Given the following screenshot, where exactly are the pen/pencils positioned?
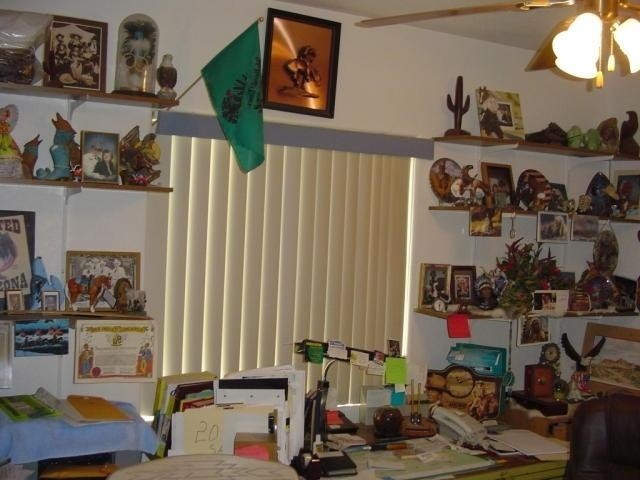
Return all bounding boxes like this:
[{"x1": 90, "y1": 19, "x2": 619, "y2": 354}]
[
  {"x1": 417, "y1": 382, "x2": 422, "y2": 423},
  {"x1": 411, "y1": 379, "x2": 415, "y2": 423}
]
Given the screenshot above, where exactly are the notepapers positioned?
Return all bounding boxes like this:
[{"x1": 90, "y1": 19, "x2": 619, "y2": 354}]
[
  {"x1": 307, "y1": 344, "x2": 324, "y2": 364},
  {"x1": 384, "y1": 389, "x2": 392, "y2": 407},
  {"x1": 365, "y1": 390, "x2": 385, "y2": 408},
  {"x1": 368, "y1": 361, "x2": 385, "y2": 376},
  {"x1": 374, "y1": 351, "x2": 385, "y2": 367},
  {"x1": 446, "y1": 314, "x2": 471, "y2": 339},
  {"x1": 327, "y1": 410, "x2": 345, "y2": 426},
  {"x1": 233, "y1": 444, "x2": 270, "y2": 463},
  {"x1": 392, "y1": 387, "x2": 405, "y2": 406},
  {"x1": 350, "y1": 350, "x2": 369, "y2": 368},
  {"x1": 385, "y1": 357, "x2": 411, "y2": 384},
  {"x1": 395, "y1": 384, "x2": 406, "y2": 393},
  {"x1": 328, "y1": 340, "x2": 348, "y2": 360}
]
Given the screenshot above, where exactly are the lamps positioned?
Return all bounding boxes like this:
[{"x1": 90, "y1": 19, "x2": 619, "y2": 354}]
[
  {"x1": 550, "y1": 13, "x2": 605, "y2": 89},
  {"x1": 608, "y1": 18, "x2": 640, "y2": 77}
]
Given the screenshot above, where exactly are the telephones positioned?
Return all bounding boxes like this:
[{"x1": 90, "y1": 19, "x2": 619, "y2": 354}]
[{"x1": 431, "y1": 404, "x2": 488, "y2": 446}]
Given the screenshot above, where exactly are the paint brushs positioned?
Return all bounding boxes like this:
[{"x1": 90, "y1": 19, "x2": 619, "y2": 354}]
[{"x1": 363, "y1": 442, "x2": 407, "y2": 452}]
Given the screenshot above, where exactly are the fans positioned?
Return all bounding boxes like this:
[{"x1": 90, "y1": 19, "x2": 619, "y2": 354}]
[{"x1": 353, "y1": 0, "x2": 640, "y2": 72}]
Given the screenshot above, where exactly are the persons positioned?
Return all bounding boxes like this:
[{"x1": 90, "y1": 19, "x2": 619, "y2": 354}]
[{"x1": 83, "y1": 142, "x2": 115, "y2": 182}]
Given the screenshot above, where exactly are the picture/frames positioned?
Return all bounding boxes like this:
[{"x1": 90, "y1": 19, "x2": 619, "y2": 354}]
[
  {"x1": 580, "y1": 322, "x2": 640, "y2": 395},
  {"x1": 81, "y1": 128, "x2": 120, "y2": 185},
  {"x1": 41, "y1": 15, "x2": 108, "y2": 94},
  {"x1": 469, "y1": 206, "x2": 502, "y2": 237},
  {"x1": 262, "y1": 6, "x2": 342, "y2": 119},
  {"x1": 419, "y1": 263, "x2": 451, "y2": 308},
  {"x1": 536, "y1": 211, "x2": 569, "y2": 244},
  {"x1": 450, "y1": 265, "x2": 477, "y2": 304},
  {"x1": 516, "y1": 313, "x2": 550, "y2": 347},
  {"x1": 475, "y1": 88, "x2": 525, "y2": 146},
  {"x1": 65, "y1": 250, "x2": 141, "y2": 313},
  {"x1": 481, "y1": 161, "x2": 515, "y2": 208},
  {"x1": 613, "y1": 169, "x2": 640, "y2": 220},
  {"x1": 531, "y1": 289, "x2": 569, "y2": 315}
]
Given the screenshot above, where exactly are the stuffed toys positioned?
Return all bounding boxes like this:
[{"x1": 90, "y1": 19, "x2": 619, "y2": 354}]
[{"x1": 65, "y1": 274, "x2": 112, "y2": 312}]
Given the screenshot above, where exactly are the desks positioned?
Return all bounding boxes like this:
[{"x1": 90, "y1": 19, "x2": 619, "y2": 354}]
[
  {"x1": 0, "y1": 400, "x2": 161, "y2": 480},
  {"x1": 301, "y1": 423, "x2": 569, "y2": 479}
]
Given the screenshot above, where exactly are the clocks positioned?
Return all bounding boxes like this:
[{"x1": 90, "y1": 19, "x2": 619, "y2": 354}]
[
  {"x1": 541, "y1": 343, "x2": 561, "y2": 362},
  {"x1": 424, "y1": 364, "x2": 502, "y2": 420}
]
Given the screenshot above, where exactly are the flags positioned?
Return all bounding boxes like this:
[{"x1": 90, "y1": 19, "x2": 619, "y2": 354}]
[{"x1": 200, "y1": 16, "x2": 266, "y2": 174}]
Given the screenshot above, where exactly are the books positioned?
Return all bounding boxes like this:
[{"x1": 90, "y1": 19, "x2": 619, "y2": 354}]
[
  {"x1": 145, "y1": 364, "x2": 307, "y2": 465},
  {"x1": 315, "y1": 451, "x2": 358, "y2": 477}
]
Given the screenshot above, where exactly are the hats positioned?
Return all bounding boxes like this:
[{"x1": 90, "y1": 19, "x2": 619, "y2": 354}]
[
  {"x1": 71, "y1": 30, "x2": 83, "y2": 39},
  {"x1": 112, "y1": 260, "x2": 122, "y2": 265},
  {"x1": 56, "y1": 33, "x2": 64, "y2": 39},
  {"x1": 91, "y1": 257, "x2": 99, "y2": 262},
  {"x1": 99, "y1": 261, "x2": 107, "y2": 266},
  {"x1": 57, "y1": 43, "x2": 67, "y2": 48}
]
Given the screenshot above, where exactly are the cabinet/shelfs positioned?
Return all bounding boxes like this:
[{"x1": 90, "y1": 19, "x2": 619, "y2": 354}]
[
  {"x1": 0, "y1": 313, "x2": 154, "y2": 322},
  {"x1": 0, "y1": 178, "x2": 173, "y2": 193},
  {"x1": 432, "y1": 136, "x2": 613, "y2": 158},
  {"x1": 413, "y1": 308, "x2": 490, "y2": 320},
  {"x1": 0, "y1": 81, "x2": 180, "y2": 120},
  {"x1": 429, "y1": 205, "x2": 612, "y2": 220}
]
